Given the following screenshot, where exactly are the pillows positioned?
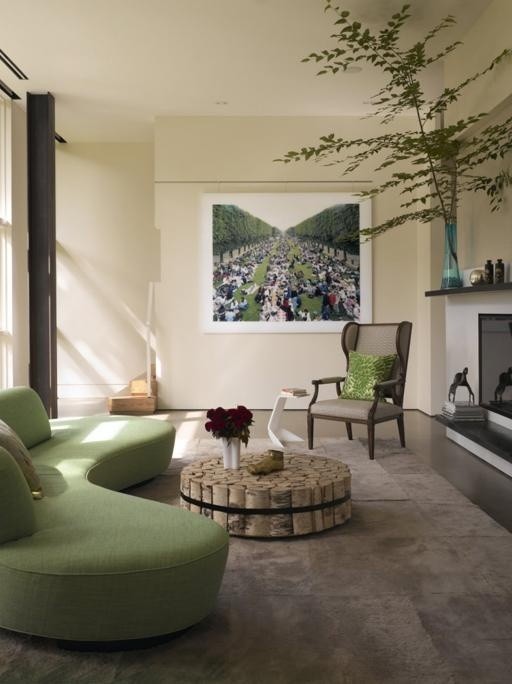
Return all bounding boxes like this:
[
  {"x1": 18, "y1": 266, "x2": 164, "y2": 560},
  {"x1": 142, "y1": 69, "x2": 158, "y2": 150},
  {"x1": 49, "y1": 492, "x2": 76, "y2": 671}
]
[
  {"x1": 2, "y1": 419, "x2": 45, "y2": 548},
  {"x1": 338, "y1": 349, "x2": 396, "y2": 403}
]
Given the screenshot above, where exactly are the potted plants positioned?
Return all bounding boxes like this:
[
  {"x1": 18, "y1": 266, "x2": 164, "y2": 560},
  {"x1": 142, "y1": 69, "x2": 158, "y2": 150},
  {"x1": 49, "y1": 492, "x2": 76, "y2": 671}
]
[{"x1": 270, "y1": 0, "x2": 512, "y2": 291}]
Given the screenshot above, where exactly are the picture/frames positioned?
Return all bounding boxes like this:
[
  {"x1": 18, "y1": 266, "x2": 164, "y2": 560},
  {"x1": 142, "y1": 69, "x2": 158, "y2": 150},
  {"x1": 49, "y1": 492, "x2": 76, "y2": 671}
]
[{"x1": 199, "y1": 191, "x2": 374, "y2": 334}]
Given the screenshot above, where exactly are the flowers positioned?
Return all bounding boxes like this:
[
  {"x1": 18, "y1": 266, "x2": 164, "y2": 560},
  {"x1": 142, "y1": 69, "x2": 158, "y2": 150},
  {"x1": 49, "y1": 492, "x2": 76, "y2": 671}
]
[{"x1": 205, "y1": 405, "x2": 254, "y2": 447}]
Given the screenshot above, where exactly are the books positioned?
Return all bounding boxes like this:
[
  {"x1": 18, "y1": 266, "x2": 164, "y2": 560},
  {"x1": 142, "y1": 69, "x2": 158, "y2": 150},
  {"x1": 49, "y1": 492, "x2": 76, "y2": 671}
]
[{"x1": 441, "y1": 401, "x2": 487, "y2": 422}]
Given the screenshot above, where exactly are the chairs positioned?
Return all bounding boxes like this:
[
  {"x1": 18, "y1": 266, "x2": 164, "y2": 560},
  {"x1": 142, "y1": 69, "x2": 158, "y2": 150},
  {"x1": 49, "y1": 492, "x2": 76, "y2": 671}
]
[{"x1": 306, "y1": 320, "x2": 412, "y2": 460}]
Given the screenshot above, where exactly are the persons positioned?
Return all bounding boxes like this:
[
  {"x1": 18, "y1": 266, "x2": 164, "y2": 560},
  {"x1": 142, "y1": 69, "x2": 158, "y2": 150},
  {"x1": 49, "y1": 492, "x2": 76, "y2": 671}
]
[{"x1": 213, "y1": 238, "x2": 360, "y2": 321}]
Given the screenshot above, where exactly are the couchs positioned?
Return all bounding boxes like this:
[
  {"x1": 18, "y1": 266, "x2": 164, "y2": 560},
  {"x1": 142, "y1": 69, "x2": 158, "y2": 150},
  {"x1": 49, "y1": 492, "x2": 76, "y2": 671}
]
[{"x1": 1, "y1": 383, "x2": 231, "y2": 651}]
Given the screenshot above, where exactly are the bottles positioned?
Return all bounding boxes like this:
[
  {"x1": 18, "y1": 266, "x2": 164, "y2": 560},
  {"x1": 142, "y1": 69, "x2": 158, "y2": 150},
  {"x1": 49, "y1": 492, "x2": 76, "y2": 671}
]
[
  {"x1": 495, "y1": 259, "x2": 504, "y2": 284},
  {"x1": 485, "y1": 260, "x2": 493, "y2": 284}
]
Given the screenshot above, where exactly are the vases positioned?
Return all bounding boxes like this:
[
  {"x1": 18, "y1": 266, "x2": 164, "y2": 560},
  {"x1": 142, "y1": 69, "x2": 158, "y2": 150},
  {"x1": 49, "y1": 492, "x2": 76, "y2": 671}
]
[{"x1": 222, "y1": 437, "x2": 240, "y2": 469}]
